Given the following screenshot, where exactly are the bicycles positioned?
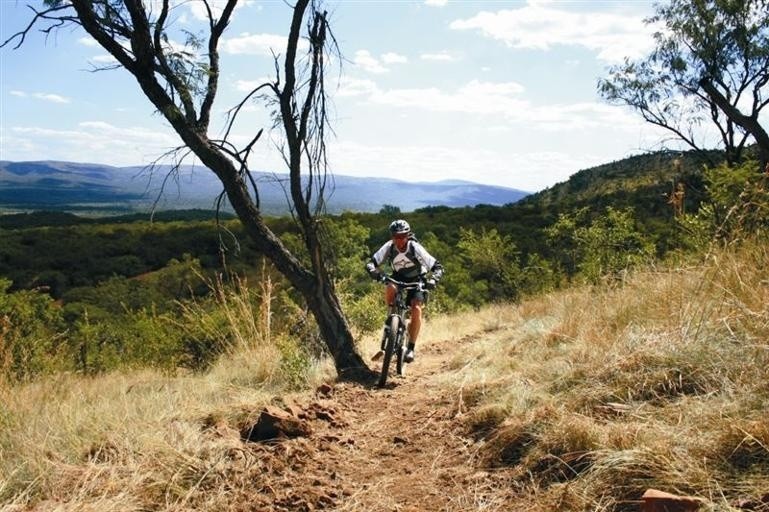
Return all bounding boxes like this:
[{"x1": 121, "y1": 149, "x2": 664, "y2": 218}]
[{"x1": 367, "y1": 270, "x2": 439, "y2": 387}]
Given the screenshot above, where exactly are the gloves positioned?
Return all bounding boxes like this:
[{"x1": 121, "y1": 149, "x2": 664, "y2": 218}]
[{"x1": 425, "y1": 278, "x2": 436, "y2": 290}]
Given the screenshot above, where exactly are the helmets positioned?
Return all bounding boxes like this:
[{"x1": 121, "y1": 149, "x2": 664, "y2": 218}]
[{"x1": 389, "y1": 220, "x2": 411, "y2": 235}]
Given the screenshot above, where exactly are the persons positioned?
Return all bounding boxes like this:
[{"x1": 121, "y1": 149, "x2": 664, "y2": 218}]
[{"x1": 365, "y1": 219, "x2": 445, "y2": 364}]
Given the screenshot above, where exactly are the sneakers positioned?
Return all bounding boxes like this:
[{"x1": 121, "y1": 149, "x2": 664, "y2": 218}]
[
  {"x1": 370, "y1": 270, "x2": 381, "y2": 280},
  {"x1": 404, "y1": 350, "x2": 414, "y2": 364}
]
